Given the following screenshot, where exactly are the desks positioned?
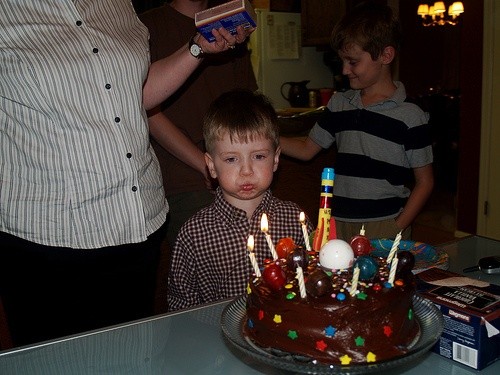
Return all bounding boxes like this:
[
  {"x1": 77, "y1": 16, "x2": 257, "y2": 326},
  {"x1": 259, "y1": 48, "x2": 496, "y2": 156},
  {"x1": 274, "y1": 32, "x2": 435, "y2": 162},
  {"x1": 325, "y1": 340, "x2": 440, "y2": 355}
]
[
  {"x1": 277, "y1": 110, "x2": 334, "y2": 226},
  {"x1": 0, "y1": 234, "x2": 500, "y2": 375}
]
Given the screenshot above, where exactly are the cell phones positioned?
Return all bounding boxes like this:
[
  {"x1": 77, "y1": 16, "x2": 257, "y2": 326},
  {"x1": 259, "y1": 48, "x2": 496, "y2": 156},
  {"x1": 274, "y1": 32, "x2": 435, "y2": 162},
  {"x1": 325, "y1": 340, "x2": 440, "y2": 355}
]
[{"x1": 463, "y1": 256, "x2": 500, "y2": 275}]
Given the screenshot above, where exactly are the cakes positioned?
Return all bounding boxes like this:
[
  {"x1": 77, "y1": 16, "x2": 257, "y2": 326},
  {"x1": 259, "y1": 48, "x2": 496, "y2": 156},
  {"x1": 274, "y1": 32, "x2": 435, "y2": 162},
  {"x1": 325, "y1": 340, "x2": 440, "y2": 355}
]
[{"x1": 244, "y1": 218, "x2": 419, "y2": 366}]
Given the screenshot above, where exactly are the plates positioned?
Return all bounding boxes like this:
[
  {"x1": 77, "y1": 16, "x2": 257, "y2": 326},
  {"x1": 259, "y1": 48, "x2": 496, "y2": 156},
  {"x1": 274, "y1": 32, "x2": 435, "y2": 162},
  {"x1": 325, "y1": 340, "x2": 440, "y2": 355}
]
[
  {"x1": 220, "y1": 291, "x2": 444, "y2": 375},
  {"x1": 411, "y1": 245, "x2": 449, "y2": 274},
  {"x1": 371, "y1": 239, "x2": 436, "y2": 269}
]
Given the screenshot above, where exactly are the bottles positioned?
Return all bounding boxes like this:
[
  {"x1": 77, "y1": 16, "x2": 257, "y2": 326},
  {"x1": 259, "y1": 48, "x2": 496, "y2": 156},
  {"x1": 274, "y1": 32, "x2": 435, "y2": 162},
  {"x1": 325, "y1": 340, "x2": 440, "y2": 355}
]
[{"x1": 463, "y1": 256, "x2": 500, "y2": 275}]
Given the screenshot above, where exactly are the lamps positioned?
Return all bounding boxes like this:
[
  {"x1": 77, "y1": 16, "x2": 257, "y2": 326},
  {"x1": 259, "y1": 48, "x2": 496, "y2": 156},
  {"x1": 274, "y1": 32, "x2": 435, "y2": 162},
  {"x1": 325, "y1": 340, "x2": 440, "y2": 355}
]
[{"x1": 418, "y1": 0, "x2": 465, "y2": 27}]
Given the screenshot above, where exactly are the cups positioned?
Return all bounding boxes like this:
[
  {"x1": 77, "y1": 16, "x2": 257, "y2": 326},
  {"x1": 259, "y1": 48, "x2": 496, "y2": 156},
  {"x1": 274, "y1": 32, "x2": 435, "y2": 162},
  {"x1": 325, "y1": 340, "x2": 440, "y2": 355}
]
[
  {"x1": 319, "y1": 88, "x2": 333, "y2": 106},
  {"x1": 308, "y1": 89, "x2": 319, "y2": 107}
]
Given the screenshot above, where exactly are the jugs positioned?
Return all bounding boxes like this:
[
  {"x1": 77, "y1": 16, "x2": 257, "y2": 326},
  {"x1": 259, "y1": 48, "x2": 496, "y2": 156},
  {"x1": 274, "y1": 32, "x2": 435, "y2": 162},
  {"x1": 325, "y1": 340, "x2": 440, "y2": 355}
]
[{"x1": 280, "y1": 80, "x2": 311, "y2": 108}]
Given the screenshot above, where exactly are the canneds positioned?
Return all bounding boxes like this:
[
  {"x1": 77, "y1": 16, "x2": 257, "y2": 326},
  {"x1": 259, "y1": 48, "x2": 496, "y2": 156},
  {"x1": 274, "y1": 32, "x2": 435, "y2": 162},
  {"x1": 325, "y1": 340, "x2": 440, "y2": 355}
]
[{"x1": 309, "y1": 91, "x2": 316, "y2": 107}]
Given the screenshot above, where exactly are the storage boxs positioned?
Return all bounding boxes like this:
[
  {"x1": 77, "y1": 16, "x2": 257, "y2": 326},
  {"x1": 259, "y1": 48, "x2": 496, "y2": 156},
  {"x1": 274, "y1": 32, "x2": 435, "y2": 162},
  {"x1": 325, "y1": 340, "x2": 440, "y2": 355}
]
[
  {"x1": 416, "y1": 276, "x2": 500, "y2": 372},
  {"x1": 193, "y1": 0, "x2": 259, "y2": 40}
]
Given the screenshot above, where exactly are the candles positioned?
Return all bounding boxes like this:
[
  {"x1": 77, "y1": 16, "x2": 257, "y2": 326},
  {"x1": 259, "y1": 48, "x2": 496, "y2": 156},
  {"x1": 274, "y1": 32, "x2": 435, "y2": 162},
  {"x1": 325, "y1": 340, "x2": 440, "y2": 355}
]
[
  {"x1": 298, "y1": 210, "x2": 312, "y2": 252},
  {"x1": 349, "y1": 263, "x2": 360, "y2": 295},
  {"x1": 388, "y1": 252, "x2": 398, "y2": 283},
  {"x1": 247, "y1": 236, "x2": 261, "y2": 278},
  {"x1": 260, "y1": 213, "x2": 279, "y2": 260},
  {"x1": 293, "y1": 258, "x2": 307, "y2": 298},
  {"x1": 387, "y1": 230, "x2": 402, "y2": 263},
  {"x1": 359, "y1": 224, "x2": 367, "y2": 237}
]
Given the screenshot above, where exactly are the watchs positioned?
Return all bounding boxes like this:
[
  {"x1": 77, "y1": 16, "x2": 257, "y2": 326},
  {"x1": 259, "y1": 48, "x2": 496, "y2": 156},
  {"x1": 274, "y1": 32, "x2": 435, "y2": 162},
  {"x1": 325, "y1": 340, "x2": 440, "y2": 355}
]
[{"x1": 189, "y1": 33, "x2": 208, "y2": 58}]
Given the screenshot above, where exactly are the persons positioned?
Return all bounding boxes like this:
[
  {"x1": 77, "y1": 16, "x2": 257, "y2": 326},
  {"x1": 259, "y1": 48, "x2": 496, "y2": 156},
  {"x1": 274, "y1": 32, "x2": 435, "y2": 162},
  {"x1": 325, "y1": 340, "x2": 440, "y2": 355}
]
[
  {"x1": 167, "y1": 90, "x2": 315, "y2": 316},
  {"x1": 0, "y1": 0, "x2": 255, "y2": 346},
  {"x1": 139, "y1": 0, "x2": 258, "y2": 251},
  {"x1": 279, "y1": 5, "x2": 436, "y2": 244}
]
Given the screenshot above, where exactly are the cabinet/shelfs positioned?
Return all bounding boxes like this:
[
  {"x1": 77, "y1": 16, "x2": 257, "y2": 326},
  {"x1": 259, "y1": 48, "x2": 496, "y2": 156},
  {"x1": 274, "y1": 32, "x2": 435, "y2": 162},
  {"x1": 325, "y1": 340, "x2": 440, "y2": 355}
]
[{"x1": 270, "y1": 0, "x2": 399, "y2": 42}]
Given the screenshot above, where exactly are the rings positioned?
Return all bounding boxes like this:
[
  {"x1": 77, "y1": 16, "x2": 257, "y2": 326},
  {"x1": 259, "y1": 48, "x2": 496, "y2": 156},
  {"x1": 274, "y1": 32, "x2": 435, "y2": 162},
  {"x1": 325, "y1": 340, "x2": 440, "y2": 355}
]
[{"x1": 227, "y1": 42, "x2": 236, "y2": 49}]
